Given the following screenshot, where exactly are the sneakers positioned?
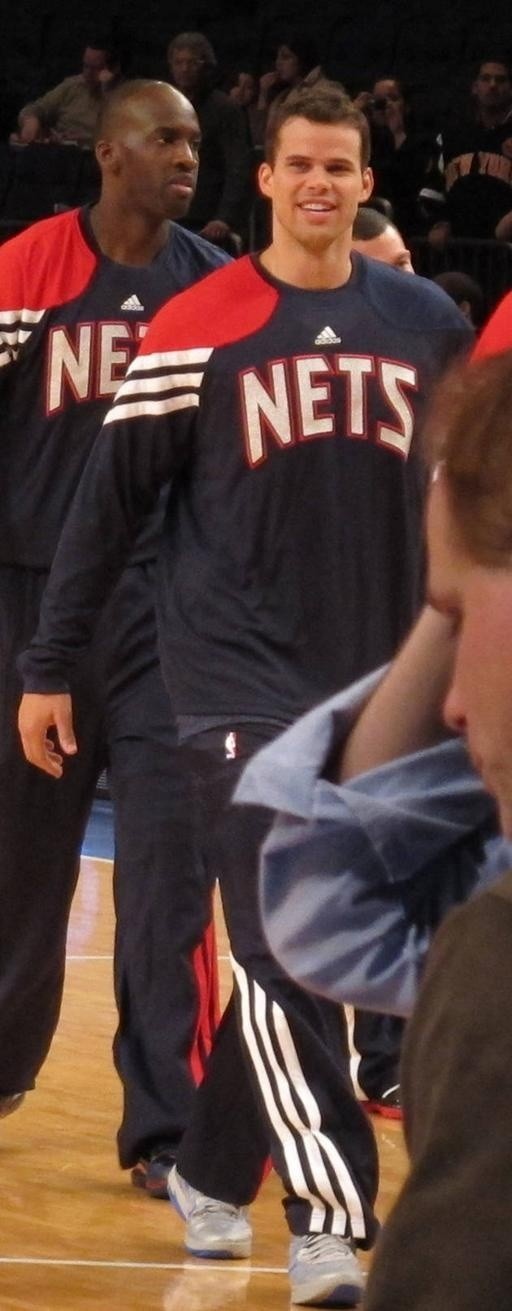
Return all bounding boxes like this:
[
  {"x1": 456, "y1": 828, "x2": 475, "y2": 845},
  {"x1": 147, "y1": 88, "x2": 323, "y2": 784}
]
[
  {"x1": 286, "y1": 1233, "x2": 364, "y2": 1307},
  {"x1": 360, "y1": 1078, "x2": 404, "y2": 1120},
  {"x1": 131, "y1": 1142, "x2": 176, "y2": 1200},
  {"x1": 165, "y1": 1163, "x2": 253, "y2": 1259}
]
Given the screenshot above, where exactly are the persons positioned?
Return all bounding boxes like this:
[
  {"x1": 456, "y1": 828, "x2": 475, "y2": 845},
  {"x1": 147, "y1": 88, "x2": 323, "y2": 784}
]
[
  {"x1": 0, "y1": 1, "x2": 512, "y2": 326},
  {"x1": 248, "y1": 570, "x2": 512, "y2": 1023},
  {"x1": 2, "y1": 71, "x2": 239, "y2": 1204},
  {"x1": 18, "y1": 81, "x2": 476, "y2": 1311},
  {"x1": 347, "y1": 341, "x2": 510, "y2": 1311}
]
